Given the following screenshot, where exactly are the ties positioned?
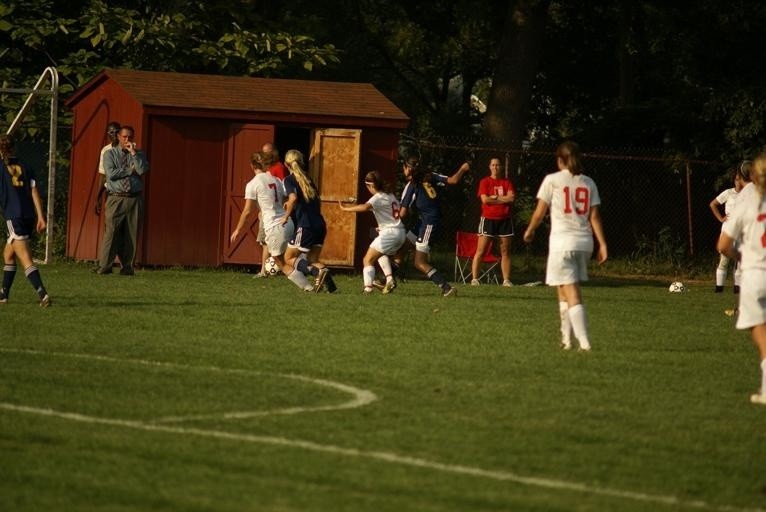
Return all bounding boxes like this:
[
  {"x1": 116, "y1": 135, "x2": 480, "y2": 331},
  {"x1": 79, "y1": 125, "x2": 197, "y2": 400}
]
[{"x1": 122, "y1": 151, "x2": 131, "y2": 192}]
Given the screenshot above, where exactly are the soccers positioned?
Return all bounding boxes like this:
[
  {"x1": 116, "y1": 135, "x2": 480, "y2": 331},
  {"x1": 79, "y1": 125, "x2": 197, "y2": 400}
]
[
  {"x1": 264, "y1": 257, "x2": 281, "y2": 276},
  {"x1": 669, "y1": 281, "x2": 685, "y2": 294}
]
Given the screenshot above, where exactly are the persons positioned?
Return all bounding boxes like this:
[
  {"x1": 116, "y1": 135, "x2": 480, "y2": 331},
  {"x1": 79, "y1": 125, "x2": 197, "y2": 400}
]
[
  {"x1": 281, "y1": 148, "x2": 338, "y2": 293},
  {"x1": 253, "y1": 143, "x2": 292, "y2": 280},
  {"x1": 92, "y1": 121, "x2": 123, "y2": 232},
  {"x1": 523, "y1": 141, "x2": 609, "y2": 352},
  {"x1": 338, "y1": 170, "x2": 408, "y2": 296},
  {"x1": 715, "y1": 159, "x2": 751, "y2": 301},
  {"x1": 1, "y1": 132, "x2": 53, "y2": 309},
  {"x1": 470, "y1": 154, "x2": 517, "y2": 287},
  {"x1": 388, "y1": 156, "x2": 473, "y2": 298},
  {"x1": 97, "y1": 125, "x2": 149, "y2": 276},
  {"x1": 733, "y1": 151, "x2": 766, "y2": 404},
  {"x1": 708, "y1": 162, "x2": 743, "y2": 295},
  {"x1": 228, "y1": 150, "x2": 316, "y2": 292}
]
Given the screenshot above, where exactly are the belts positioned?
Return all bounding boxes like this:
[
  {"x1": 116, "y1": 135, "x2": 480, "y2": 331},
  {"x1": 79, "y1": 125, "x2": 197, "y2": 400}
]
[{"x1": 109, "y1": 192, "x2": 135, "y2": 197}]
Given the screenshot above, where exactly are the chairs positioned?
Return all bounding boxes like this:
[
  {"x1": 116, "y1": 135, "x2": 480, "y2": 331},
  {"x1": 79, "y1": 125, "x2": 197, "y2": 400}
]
[{"x1": 454, "y1": 231, "x2": 499, "y2": 285}]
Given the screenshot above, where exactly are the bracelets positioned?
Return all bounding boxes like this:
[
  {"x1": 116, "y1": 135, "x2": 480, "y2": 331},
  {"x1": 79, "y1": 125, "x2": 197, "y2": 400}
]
[{"x1": 131, "y1": 153, "x2": 136, "y2": 157}]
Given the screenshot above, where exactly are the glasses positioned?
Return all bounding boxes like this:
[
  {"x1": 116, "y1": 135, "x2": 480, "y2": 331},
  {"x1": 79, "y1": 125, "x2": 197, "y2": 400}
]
[{"x1": 107, "y1": 130, "x2": 119, "y2": 135}]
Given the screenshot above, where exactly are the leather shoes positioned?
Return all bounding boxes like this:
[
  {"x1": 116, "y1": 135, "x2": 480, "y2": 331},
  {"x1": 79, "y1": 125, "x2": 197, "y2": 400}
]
[
  {"x1": 96, "y1": 267, "x2": 111, "y2": 275},
  {"x1": 120, "y1": 266, "x2": 133, "y2": 275}
]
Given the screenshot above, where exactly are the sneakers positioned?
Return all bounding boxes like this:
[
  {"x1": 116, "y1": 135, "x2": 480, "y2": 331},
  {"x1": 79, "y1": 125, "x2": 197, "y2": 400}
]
[
  {"x1": 39, "y1": 292, "x2": 50, "y2": 307},
  {"x1": 470, "y1": 279, "x2": 479, "y2": 286},
  {"x1": 502, "y1": 280, "x2": 512, "y2": 287},
  {"x1": 0, "y1": 287, "x2": 8, "y2": 304},
  {"x1": 441, "y1": 283, "x2": 456, "y2": 297},
  {"x1": 303, "y1": 267, "x2": 338, "y2": 294},
  {"x1": 381, "y1": 279, "x2": 396, "y2": 294},
  {"x1": 360, "y1": 285, "x2": 372, "y2": 294},
  {"x1": 373, "y1": 279, "x2": 385, "y2": 290},
  {"x1": 251, "y1": 272, "x2": 267, "y2": 279},
  {"x1": 750, "y1": 393, "x2": 766, "y2": 405}
]
